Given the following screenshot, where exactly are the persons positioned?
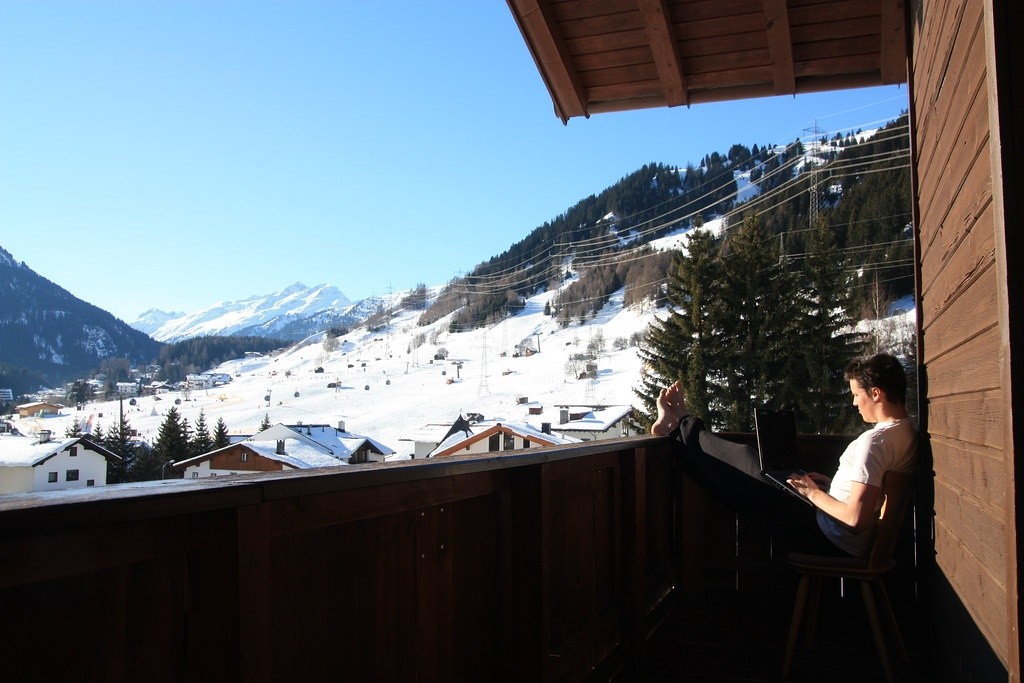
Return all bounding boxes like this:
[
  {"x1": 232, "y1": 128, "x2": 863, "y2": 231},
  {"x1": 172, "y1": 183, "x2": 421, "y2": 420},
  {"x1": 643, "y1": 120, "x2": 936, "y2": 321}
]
[{"x1": 651, "y1": 353, "x2": 920, "y2": 556}]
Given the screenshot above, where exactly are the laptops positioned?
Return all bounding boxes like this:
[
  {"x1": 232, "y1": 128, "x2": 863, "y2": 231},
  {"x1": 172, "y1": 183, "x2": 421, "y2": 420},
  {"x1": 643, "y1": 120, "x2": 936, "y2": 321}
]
[{"x1": 754, "y1": 407, "x2": 815, "y2": 506}]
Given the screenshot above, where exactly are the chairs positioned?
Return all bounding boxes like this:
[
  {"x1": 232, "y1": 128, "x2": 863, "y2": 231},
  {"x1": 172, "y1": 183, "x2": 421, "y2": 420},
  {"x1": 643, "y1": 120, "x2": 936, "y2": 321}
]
[{"x1": 781, "y1": 470, "x2": 919, "y2": 683}]
